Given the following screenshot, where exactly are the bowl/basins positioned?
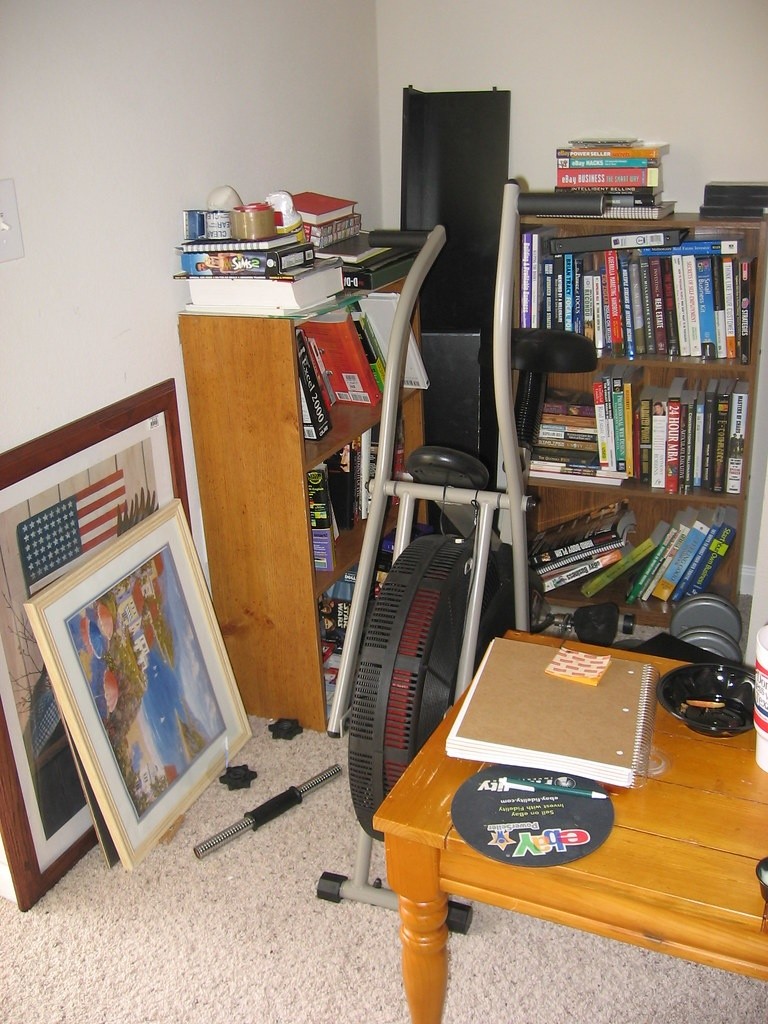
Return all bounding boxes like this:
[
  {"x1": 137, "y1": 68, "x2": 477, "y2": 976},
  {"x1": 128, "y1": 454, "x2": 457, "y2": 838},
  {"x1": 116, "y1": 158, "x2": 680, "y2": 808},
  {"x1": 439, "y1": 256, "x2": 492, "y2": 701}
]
[{"x1": 656, "y1": 663, "x2": 759, "y2": 738}]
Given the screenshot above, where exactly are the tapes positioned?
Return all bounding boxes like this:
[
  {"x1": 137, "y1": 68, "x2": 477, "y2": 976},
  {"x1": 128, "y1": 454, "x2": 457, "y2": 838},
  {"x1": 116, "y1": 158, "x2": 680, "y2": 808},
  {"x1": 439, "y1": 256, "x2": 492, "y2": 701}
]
[
  {"x1": 230, "y1": 202, "x2": 277, "y2": 241},
  {"x1": 182, "y1": 207, "x2": 231, "y2": 240}
]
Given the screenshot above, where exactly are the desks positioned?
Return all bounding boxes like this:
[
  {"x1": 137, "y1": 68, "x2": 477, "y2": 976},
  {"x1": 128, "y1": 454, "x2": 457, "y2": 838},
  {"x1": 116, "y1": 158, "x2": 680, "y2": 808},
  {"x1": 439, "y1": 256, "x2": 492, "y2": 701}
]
[{"x1": 373, "y1": 630, "x2": 768, "y2": 1024}]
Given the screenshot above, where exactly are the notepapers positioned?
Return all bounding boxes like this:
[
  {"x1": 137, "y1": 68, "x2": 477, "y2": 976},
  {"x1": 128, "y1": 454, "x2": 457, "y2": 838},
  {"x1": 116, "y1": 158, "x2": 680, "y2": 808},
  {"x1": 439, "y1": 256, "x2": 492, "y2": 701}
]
[{"x1": 544, "y1": 647, "x2": 612, "y2": 687}]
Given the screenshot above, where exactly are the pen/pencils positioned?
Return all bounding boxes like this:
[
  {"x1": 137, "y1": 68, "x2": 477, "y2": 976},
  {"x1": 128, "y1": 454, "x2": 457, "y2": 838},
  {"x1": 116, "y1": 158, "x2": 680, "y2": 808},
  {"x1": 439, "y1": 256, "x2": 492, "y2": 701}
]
[{"x1": 498, "y1": 777, "x2": 609, "y2": 800}]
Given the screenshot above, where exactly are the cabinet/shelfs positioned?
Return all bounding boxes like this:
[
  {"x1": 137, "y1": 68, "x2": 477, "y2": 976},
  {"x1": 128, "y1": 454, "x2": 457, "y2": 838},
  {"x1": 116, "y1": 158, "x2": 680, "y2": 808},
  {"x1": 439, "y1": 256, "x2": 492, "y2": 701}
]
[
  {"x1": 510, "y1": 208, "x2": 768, "y2": 650},
  {"x1": 180, "y1": 271, "x2": 427, "y2": 734}
]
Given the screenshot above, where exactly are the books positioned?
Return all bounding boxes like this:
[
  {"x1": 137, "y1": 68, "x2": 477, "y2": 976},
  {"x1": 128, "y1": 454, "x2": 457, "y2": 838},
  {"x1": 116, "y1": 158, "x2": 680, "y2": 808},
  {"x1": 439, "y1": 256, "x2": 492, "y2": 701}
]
[{"x1": 177, "y1": 137, "x2": 756, "y2": 793}]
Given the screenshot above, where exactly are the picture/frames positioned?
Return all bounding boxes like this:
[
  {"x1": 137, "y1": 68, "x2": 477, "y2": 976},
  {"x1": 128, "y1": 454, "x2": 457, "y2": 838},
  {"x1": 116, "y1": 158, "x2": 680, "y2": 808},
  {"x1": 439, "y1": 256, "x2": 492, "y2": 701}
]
[
  {"x1": 0, "y1": 377, "x2": 194, "y2": 912},
  {"x1": 22, "y1": 496, "x2": 253, "y2": 872}
]
